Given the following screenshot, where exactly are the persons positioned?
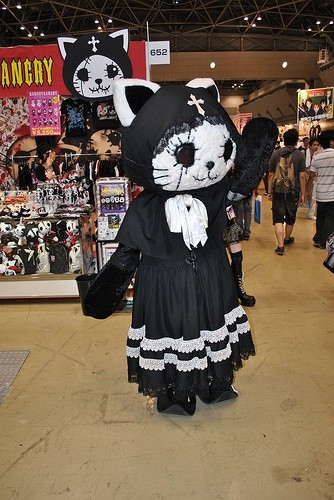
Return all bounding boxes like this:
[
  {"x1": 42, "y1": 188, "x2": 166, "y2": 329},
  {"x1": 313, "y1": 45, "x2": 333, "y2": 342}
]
[{"x1": 223, "y1": 130, "x2": 334, "y2": 307}]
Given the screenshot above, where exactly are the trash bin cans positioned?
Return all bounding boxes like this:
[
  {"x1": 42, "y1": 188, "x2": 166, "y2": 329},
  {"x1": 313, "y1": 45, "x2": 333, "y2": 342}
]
[{"x1": 75, "y1": 273, "x2": 98, "y2": 316}]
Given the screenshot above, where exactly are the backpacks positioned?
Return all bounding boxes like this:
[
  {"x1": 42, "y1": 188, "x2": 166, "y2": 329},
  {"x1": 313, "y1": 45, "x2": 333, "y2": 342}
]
[{"x1": 270, "y1": 148, "x2": 300, "y2": 196}]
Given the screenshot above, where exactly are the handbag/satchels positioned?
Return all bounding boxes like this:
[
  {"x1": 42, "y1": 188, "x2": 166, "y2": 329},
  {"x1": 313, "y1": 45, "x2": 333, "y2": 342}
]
[{"x1": 253, "y1": 196, "x2": 261, "y2": 226}]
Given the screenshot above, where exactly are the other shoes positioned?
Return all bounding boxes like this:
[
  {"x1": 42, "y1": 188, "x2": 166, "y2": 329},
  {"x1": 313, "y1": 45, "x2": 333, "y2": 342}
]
[
  {"x1": 284, "y1": 236, "x2": 294, "y2": 247},
  {"x1": 275, "y1": 246, "x2": 285, "y2": 254}
]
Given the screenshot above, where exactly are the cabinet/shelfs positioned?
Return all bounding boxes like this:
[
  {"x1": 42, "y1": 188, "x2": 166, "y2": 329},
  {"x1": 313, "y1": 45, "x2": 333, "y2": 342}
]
[{"x1": 0, "y1": 179, "x2": 96, "y2": 298}]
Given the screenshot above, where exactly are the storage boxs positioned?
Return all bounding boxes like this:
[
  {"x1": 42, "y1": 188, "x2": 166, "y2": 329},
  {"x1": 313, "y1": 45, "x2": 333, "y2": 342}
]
[
  {"x1": 97, "y1": 211, "x2": 127, "y2": 241},
  {"x1": 96, "y1": 176, "x2": 130, "y2": 212}
]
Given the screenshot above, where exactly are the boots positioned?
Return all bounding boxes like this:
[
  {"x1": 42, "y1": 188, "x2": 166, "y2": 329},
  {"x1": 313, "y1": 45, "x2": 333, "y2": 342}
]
[{"x1": 230, "y1": 271, "x2": 256, "y2": 307}]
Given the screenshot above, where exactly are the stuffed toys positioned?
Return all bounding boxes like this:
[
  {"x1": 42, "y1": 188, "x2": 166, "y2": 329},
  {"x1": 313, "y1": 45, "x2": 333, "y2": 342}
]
[
  {"x1": 81, "y1": 78, "x2": 279, "y2": 416},
  {"x1": 0, "y1": 172, "x2": 90, "y2": 276}
]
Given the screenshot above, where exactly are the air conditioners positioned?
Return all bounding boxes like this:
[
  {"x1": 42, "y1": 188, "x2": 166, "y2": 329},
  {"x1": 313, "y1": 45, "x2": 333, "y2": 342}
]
[{"x1": 316, "y1": 50, "x2": 328, "y2": 64}]
